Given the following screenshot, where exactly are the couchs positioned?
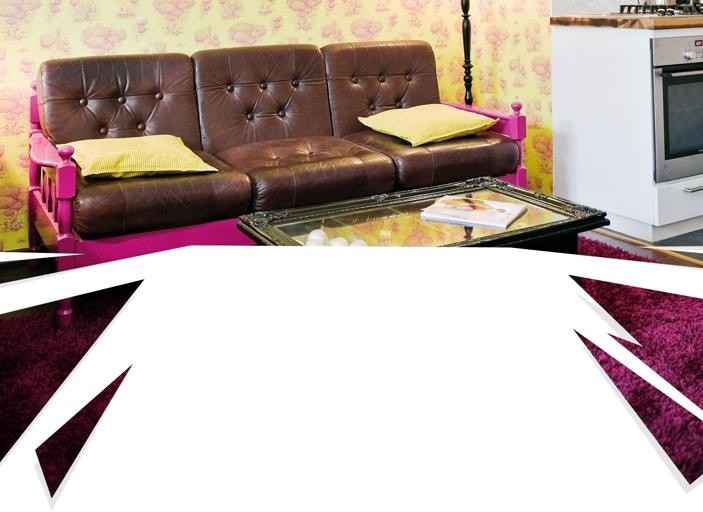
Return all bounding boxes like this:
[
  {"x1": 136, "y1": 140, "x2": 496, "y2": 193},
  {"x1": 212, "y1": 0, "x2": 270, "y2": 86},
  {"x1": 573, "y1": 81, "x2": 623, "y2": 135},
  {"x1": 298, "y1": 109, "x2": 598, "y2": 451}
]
[{"x1": 25, "y1": 40, "x2": 529, "y2": 324}]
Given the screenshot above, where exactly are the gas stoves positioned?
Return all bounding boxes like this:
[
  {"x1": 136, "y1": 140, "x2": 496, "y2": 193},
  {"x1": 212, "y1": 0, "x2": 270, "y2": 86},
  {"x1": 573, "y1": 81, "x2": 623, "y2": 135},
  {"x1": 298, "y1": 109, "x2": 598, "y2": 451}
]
[{"x1": 611, "y1": 1, "x2": 703, "y2": 16}]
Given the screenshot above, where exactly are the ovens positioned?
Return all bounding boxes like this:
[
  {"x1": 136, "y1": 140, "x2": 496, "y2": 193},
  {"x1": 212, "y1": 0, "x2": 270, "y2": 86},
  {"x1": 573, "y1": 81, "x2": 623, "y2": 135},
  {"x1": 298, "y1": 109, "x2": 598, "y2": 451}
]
[{"x1": 650, "y1": 32, "x2": 703, "y2": 185}]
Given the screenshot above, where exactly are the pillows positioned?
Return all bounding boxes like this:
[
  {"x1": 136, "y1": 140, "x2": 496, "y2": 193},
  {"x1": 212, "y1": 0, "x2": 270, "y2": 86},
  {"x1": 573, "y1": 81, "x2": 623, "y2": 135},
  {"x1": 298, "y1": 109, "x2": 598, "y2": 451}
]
[
  {"x1": 53, "y1": 132, "x2": 220, "y2": 179},
  {"x1": 354, "y1": 103, "x2": 498, "y2": 148}
]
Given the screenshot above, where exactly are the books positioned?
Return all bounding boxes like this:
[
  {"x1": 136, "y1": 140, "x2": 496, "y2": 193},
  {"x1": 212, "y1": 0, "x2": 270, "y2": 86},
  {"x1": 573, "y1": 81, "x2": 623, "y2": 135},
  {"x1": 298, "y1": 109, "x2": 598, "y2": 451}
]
[{"x1": 419, "y1": 195, "x2": 528, "y2": 229}]
